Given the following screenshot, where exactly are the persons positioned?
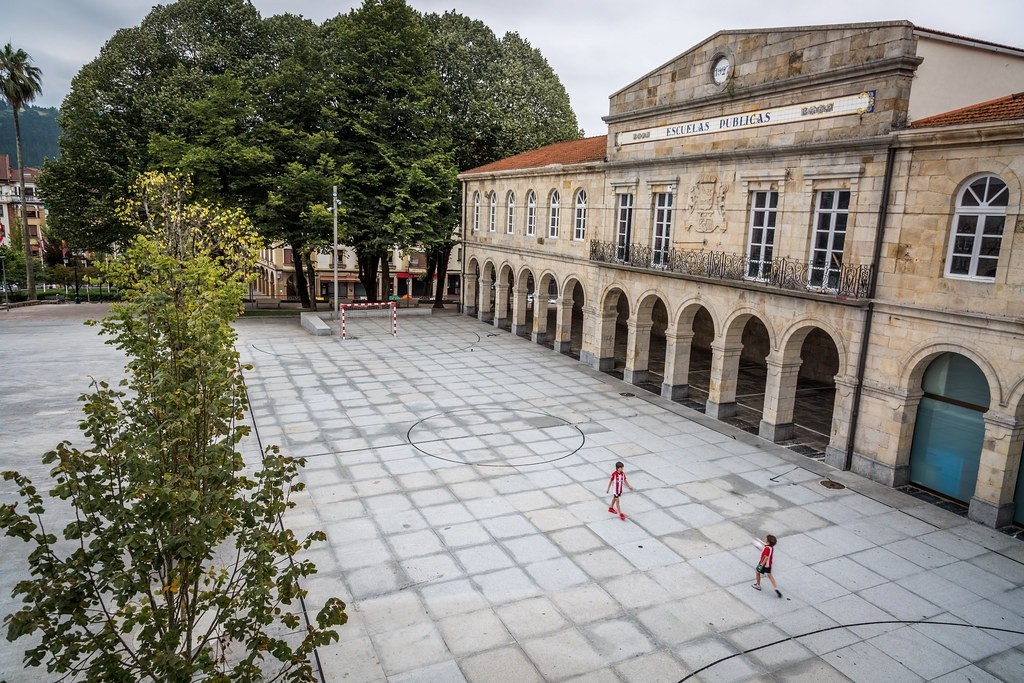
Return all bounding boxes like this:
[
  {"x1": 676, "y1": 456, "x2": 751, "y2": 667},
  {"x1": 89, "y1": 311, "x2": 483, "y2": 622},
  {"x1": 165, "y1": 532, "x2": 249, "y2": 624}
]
[
  {"x1": 751, "y1": 534, "x2": 782, "y2": 598},
  {"x1": 606, "y1": 462, "x2": 632, "y2": 519}
]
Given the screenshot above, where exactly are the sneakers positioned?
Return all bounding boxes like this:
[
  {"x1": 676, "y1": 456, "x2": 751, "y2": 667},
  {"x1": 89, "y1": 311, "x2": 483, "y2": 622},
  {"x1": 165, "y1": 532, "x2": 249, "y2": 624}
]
[
  {"x1": 620, "y1": 513, "x2": 627, "y2": 520},
  {"x1": 608, "y1": 508, "x2": 617, "y2": 514}
]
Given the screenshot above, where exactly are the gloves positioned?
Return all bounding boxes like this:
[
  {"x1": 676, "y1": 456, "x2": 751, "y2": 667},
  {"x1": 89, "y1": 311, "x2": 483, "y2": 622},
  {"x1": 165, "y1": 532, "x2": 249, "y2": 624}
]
[{"x1": 757, "y1": 564, "x2": 763, "y2": 572}]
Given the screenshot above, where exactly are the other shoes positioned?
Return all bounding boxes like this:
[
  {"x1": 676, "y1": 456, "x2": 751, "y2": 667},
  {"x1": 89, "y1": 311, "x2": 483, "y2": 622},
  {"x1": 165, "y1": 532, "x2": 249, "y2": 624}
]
[
  {"x1": 775, "y1": 590, "x2": 782, "y2": 597},
  {"x1": 752, "y1": 584, "x2": 761, "y2": 590}
]
